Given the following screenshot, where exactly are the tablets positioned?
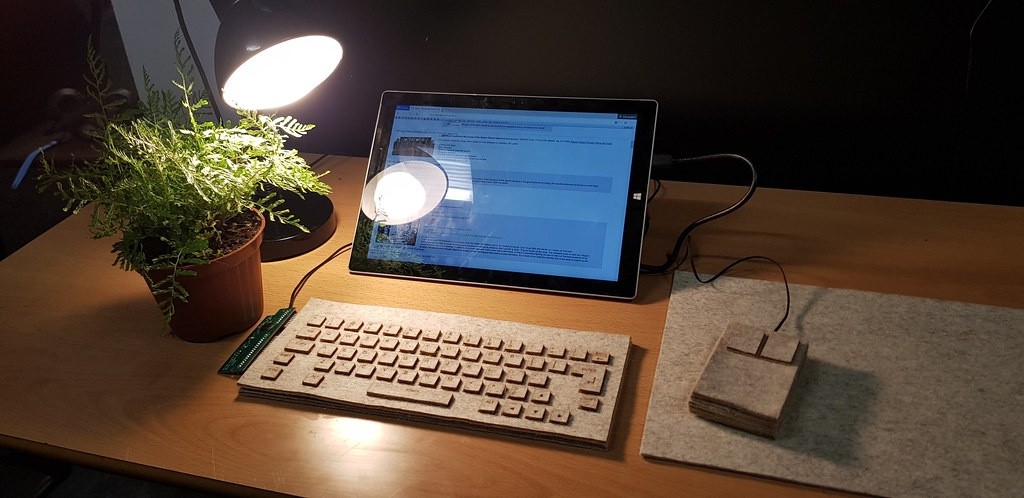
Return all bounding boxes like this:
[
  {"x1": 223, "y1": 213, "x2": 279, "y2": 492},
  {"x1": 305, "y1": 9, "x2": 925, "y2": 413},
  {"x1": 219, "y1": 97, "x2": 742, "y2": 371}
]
[{"x1": 347, "y1": 90, "x2": 658, "y2": 300}]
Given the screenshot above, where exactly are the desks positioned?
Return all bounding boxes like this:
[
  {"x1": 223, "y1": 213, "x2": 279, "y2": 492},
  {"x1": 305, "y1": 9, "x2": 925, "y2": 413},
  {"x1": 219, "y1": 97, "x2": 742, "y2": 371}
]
[{"x1": 0, "y1": 146, "x2": 1024, "y2": 498}]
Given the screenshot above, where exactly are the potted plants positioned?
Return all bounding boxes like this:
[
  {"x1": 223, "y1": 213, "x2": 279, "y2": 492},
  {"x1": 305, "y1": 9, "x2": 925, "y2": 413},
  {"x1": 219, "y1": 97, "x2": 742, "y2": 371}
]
[{"x1": 33, "y1": 25, "x2": 333, "y2": 344}]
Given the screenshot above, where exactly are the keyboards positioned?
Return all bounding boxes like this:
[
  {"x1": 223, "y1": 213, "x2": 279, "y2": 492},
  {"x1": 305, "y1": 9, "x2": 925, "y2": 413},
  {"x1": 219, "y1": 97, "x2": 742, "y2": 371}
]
[{"x1": 235, "y1": 297, "x2": 632, "y2": 452}]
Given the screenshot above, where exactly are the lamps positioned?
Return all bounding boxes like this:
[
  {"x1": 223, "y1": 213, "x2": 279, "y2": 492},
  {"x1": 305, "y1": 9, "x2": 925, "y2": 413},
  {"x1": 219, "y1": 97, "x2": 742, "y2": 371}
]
[{"x1": 173, "y1": 0, "x2": 346, "y2": 262}]
